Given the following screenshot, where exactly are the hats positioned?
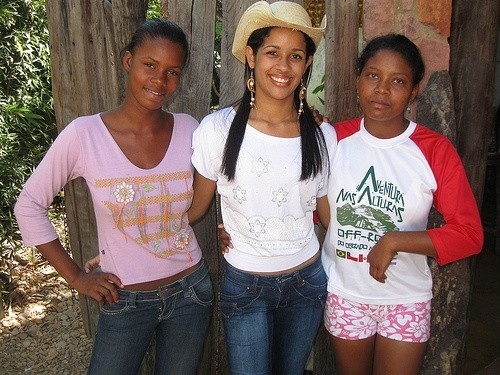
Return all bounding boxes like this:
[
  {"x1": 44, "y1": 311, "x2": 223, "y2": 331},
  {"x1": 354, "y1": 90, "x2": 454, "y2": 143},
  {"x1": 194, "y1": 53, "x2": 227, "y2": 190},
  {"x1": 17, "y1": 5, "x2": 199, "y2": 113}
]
[{"x1": 232, "y1": 1, "x2": 327, "y2": 65}]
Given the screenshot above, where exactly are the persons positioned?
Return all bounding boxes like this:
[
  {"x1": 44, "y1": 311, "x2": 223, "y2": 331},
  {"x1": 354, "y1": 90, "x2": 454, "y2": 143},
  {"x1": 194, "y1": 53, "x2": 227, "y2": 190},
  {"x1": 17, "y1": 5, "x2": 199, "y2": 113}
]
[
  {"x1": 218, "y1": 34, "x2": 483, "y2": 375},
  {"x1": 86, "y1": 1, "x2": 338, "y2": 375},
  {"x1": 14, "y1": 18, "x2": 323, "y2": 375}
]
